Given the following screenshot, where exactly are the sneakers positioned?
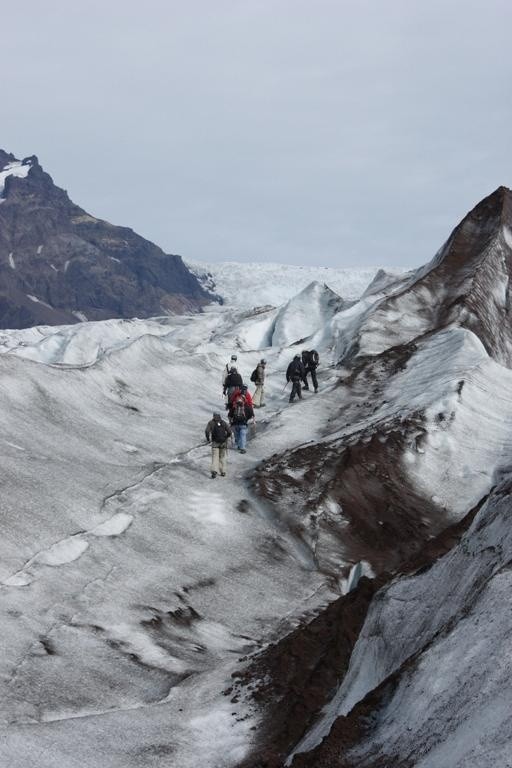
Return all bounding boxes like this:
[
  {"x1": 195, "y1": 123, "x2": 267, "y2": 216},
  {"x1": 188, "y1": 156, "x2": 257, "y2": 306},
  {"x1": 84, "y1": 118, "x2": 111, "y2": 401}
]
[
  {"x1": 253, "y1": 403, "x2": 266, "y2": 408},
  {"x1": 302, "y1": 385, "x2": 319, "y2": 393},
  {"x1": 238, "y1": 446, "x2": 246, "y2": 454},
  {"x1": 289, "y1": 397, "x2": 305, "y2": 403}
]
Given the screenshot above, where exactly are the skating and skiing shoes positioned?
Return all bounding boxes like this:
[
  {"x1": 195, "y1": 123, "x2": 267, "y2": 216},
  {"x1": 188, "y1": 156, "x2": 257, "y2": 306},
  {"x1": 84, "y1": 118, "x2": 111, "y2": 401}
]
[{"x1": 212, "y1": 471, "x2": 225, "y2": 478}]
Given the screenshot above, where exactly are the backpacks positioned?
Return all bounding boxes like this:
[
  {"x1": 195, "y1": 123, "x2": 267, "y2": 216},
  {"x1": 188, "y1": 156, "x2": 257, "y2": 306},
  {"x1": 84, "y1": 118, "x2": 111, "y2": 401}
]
[
  {"x1": 250, "y1": 366, "x2": 261, "y2": 382},
  {"x1": 229, "y1": 373, "x2": 243, "y2": 387},
  {"x1": 232, "y1": 399, "x2": 247, "y2": 421},
  {"x1": 212, "y1": 418, "x2": 228, "y2": 442},
  {"x1": 305, "y1": 350, "x2": 319, "y2": 369}
]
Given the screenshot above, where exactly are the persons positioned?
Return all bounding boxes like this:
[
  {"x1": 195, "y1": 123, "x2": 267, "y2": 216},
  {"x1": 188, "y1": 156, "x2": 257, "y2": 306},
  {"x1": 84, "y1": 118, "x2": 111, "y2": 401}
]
[
  {"x1": 285, "y1": 349, "x2": 319, "y2": 403},
  {"x1": 205, "y1": 411, "x2": 231, "y2": 478},
  {"x1": 250, "y1": 358, "x2": 267, "y2": 409},
  {"x1": 219, "y1": 354, "x2": 256, "y2": 454}
]
